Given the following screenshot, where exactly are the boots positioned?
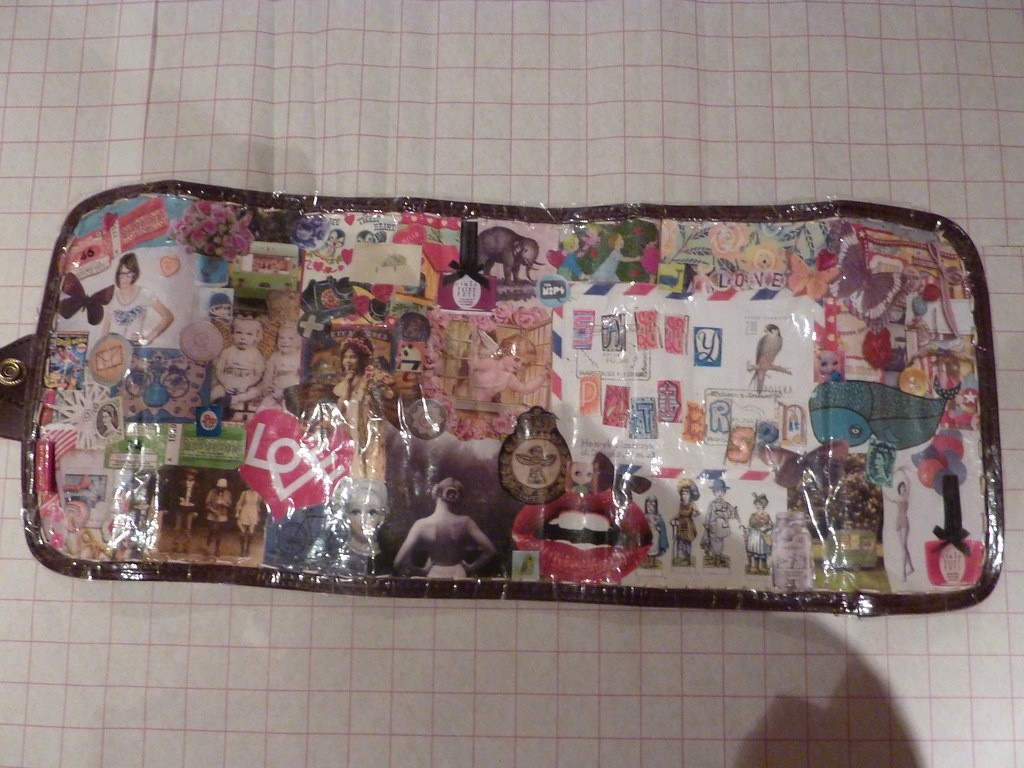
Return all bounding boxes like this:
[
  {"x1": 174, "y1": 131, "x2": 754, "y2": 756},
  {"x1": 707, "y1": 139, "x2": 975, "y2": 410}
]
[
  {"x1": 246, "y1": 534, "x2": 253, "y2": 555},
  {"x1": 240, "y1": 535, "x2": 245, "y2": 555}
]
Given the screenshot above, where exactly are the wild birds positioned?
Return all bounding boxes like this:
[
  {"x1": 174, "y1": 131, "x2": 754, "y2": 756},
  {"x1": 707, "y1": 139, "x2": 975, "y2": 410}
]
[{"x1": 755, "y1": 325, "x2": 783, "y2": 396}]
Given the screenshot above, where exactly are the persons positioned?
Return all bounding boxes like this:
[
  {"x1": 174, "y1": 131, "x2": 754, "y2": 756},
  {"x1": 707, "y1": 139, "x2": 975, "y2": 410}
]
[
  {"x1": 235, "y1": 487, "x2": 263, "y2": 556},
  {"x1": 210, "y1": 317, "x2": 265, "y2": 405},
  {"x1": 54, "y1": 343, "x2": 79, "y2": 377},
  {"x1": 102, "y1": 254, "x2": 175, "y2": 345},
  {"x1": 256, "y1": 322, "x2": 301, "y2": 414},
  {"x1": 394, "y1": 478, "x2": 496, "y2": 578},
  {"x1": 333, "y1": 346, "x2": 369, "y2": 414},
  {"x1": 171, "y1": 470, "x2": 202, "y2": 554},
  {"x1": 205, "y1": 478, "x2": 232, "y2": 555},
  {"x1": 100, "y1": 408, "x2": 117, "y2": 437}
]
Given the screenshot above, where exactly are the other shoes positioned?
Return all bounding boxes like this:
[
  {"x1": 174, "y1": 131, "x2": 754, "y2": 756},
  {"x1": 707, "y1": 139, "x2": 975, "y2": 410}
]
[
  {"x1": 215, "y1": 549, "x2": 220, "y2": 557},
  {"x1": 184, "y1": 547, "x2": 188, "y2": 553},
  {"x1": 169, "y1": 547, "x2": 176, "y2": 552}
]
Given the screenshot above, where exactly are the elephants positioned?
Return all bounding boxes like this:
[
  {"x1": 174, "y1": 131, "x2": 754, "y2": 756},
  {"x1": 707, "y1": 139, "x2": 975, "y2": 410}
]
[{"x1": 478, "y1": 226, "x2": 545, "y2": 285}]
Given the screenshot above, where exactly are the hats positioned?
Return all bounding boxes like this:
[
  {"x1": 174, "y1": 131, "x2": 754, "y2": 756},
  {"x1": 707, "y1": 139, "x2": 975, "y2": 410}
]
[
  {"x1": 187, "y1": 469, "x2": 197, "y2": 475},
  {"x1": 216, "y1": 479, "x2": 228, "y2": 488}
]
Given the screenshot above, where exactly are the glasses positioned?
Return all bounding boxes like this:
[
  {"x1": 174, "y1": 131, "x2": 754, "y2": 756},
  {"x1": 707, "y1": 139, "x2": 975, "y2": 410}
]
[{"x1": 119, "y1": 271, "x2": 134, "y2": 278}]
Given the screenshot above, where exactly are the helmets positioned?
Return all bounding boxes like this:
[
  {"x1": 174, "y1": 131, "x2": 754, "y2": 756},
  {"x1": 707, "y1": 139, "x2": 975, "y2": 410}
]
[{"x1": 208, "y1": 292, "x2": 230, "y2": 316}]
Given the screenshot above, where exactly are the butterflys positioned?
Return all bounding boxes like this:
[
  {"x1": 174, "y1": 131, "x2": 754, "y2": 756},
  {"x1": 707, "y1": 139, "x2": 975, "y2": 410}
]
[{"x1": 58, "y1": 272, "x2": 115, "y2": 326}]
